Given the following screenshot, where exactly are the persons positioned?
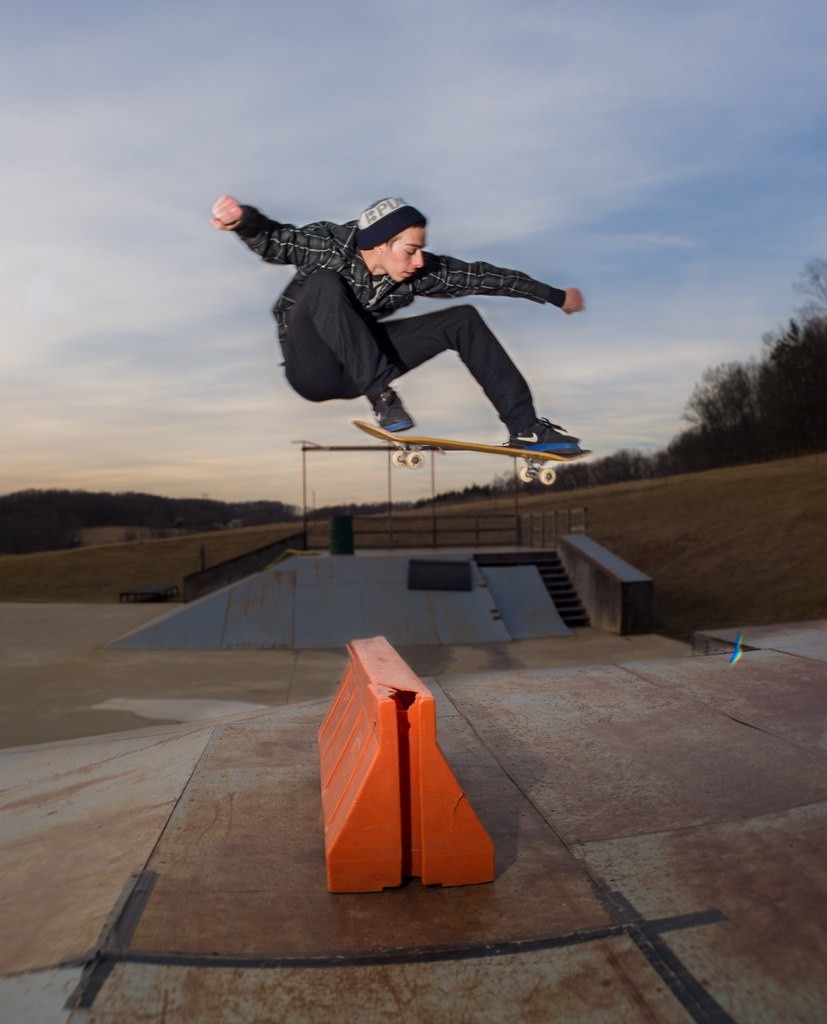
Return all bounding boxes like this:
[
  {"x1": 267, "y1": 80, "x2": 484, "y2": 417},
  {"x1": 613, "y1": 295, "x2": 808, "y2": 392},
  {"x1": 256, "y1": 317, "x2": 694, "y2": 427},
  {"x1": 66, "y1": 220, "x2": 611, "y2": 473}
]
[{"x1": 209, "y1": 195, "x2": 586, "y2": 455}]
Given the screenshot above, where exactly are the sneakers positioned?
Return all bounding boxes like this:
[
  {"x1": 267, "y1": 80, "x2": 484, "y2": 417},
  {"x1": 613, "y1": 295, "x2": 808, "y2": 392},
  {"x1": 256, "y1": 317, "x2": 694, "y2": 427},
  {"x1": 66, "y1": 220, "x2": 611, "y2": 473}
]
[
  {"x1": 372, "y1": 389, "x2": 415, "y2": 432},
  {"x1": 509, "y1": 417, "x2": 581, "y2": 452}
]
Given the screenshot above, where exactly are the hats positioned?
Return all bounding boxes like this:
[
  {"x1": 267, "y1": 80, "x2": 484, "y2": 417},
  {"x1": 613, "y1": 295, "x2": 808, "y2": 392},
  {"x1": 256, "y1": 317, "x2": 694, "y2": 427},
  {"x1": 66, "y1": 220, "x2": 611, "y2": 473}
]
[{"x1": 356, "y1": 198, "x2": 427, "y2": 250}]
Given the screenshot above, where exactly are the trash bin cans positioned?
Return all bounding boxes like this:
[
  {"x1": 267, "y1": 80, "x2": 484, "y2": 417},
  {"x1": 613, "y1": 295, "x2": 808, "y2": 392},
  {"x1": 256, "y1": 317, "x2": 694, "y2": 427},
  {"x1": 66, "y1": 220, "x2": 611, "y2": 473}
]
[{"x1": 330, "y1": 514, "x2": 355, "y2": 554}]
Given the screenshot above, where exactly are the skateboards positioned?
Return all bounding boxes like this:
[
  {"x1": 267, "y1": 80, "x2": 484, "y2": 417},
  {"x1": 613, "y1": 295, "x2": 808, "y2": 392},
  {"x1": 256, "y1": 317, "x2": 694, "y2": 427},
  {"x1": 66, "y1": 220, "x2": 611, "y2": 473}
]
[{"x1": 352, "y1": 419, "x2": 594, "y2": 486}]
